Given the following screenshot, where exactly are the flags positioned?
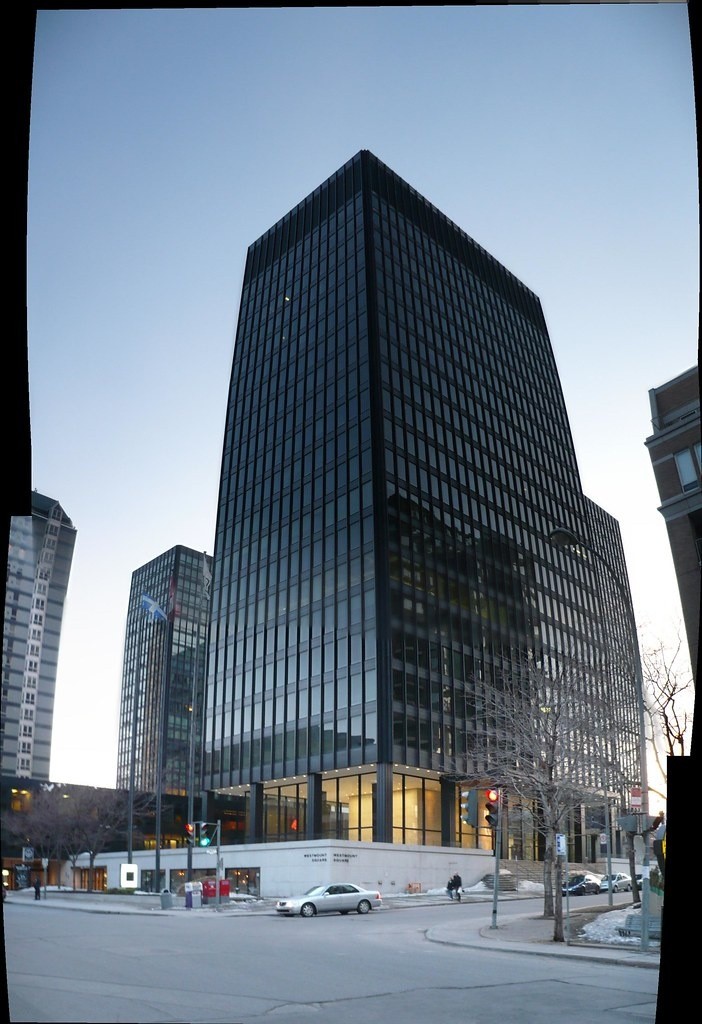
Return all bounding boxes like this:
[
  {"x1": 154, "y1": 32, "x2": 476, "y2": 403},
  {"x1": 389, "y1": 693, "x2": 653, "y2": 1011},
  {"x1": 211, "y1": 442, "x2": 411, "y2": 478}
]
[
  {"x1": 141, "y1": 592, "x2": 168, "y2": 620},
  {"x1": 202, "y1": 557, "x2": 213, "y2": 601},
  {"x1": 168, "y1": 576, "x2": 175, "y2": 623}
]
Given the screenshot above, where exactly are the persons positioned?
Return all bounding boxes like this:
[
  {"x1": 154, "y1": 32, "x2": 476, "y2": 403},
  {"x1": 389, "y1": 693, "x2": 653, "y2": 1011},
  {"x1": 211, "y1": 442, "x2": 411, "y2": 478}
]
[
  {"x1": 447, "y1": 872, "x2": 462, "y2": 902},
  {"x1": 34, "y1": 876, "x2": 41, "y2": 900}
]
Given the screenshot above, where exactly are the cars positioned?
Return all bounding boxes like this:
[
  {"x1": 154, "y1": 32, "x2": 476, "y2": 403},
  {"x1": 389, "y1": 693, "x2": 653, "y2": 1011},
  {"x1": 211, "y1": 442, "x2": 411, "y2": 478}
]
[
  {"x1": 599, "y1": 872, "x2": 632, "y2": 893},
  {"x1": 636, "y1": 873, "x2": 643, "y2": 891},
  {"x1": 275, "y1": 882, "x2": 383, "y2": 918},
  {"x1": 560, "y1": 874, "x2": 601, "y2": 897}
]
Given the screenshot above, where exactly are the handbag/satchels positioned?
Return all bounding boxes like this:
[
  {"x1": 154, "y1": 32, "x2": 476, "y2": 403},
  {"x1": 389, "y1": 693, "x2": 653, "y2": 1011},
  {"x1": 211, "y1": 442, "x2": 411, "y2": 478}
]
[{"x1": 457, "y1": 886, "x2": 462, "y2": 894}]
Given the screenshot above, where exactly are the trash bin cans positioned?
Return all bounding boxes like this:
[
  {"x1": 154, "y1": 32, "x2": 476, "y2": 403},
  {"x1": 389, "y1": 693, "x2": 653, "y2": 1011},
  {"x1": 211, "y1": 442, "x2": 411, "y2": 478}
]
[
  {"x1": 160, "y1": 889, "x2": 174, "y2": 910},
  {"x1": 184, "y1": 881, "x2": 205, "y2": 909},
  {"x1": 634, "y1": 874, "x2": 643, "y2": 891}
]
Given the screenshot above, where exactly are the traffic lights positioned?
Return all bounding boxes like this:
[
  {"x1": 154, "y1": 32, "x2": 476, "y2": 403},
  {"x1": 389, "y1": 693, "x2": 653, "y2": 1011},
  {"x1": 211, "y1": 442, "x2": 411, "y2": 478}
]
[
  {"x1": 460, "y1": 791, "x2": 478, "y2": 826},
  {"x1": 484, "y1": 788, "x2": 499, "y2": 827},
  {"x1": 199, "y1": 822, "x2": 210, "y2": 846},
  {"x1": 185, "y1": 823, "x2": 195, "y2": 847}
]
